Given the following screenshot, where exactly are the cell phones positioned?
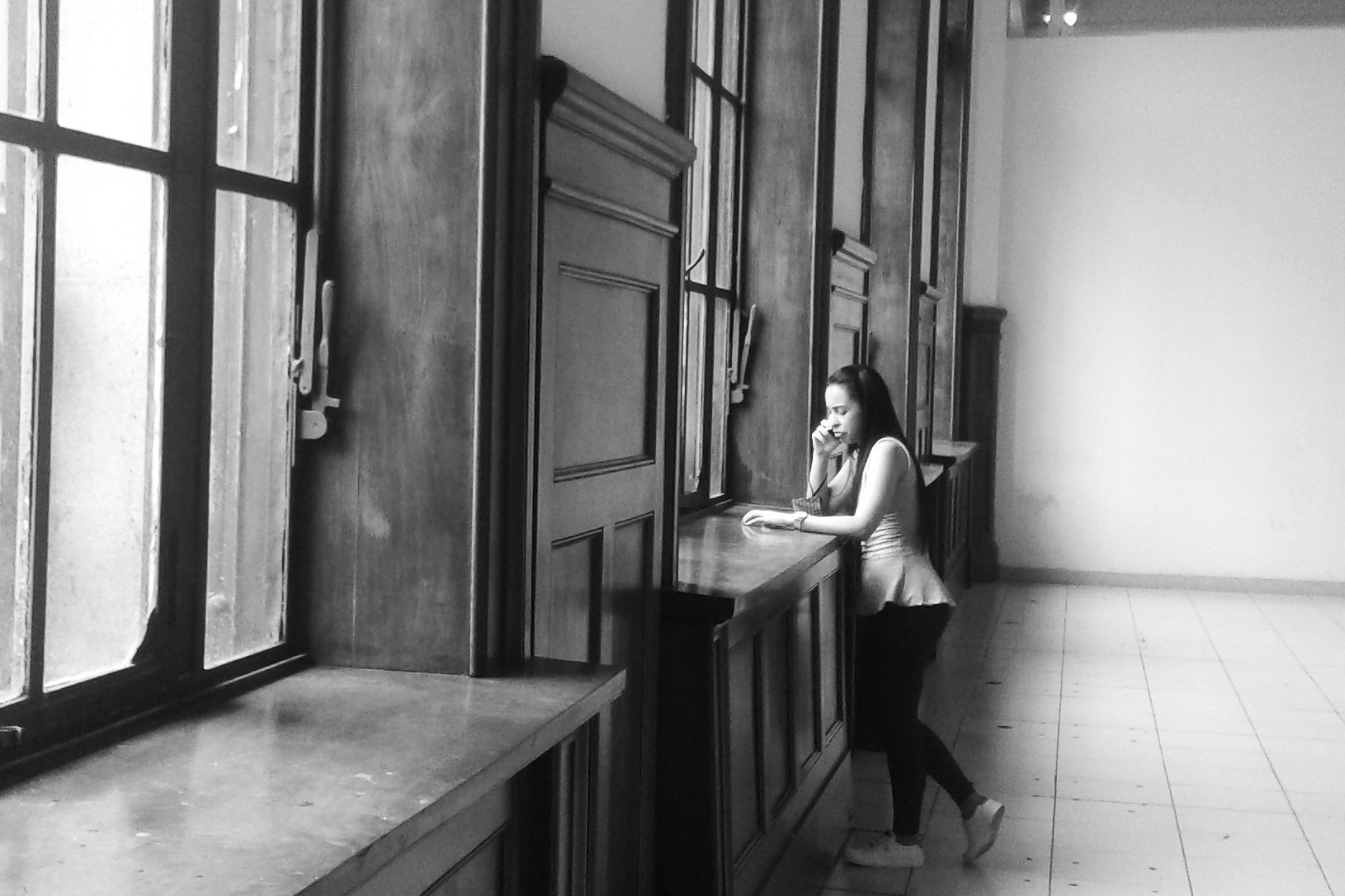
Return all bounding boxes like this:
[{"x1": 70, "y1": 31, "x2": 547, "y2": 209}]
[{"x1": 827, "y1": 428, "x2": 840, "y2": 441}]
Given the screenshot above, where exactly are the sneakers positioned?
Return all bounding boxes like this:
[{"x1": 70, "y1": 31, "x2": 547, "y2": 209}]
[
  {"x1": 962, "y1": 798, "x2": 1004, "y2": 866},
  {"x1": 845, "y1": 827, "x2": 926, "y2": 868}
]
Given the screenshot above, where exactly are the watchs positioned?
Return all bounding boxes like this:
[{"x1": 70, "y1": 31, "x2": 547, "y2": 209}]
[{"x1": 792, "y1": 510, "x2": 808, "y2": 529}]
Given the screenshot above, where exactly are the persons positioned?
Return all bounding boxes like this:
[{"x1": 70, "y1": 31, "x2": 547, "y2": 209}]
[{"x1": 739, "y1": 363, "x2": 1006, "y2": 871}]
